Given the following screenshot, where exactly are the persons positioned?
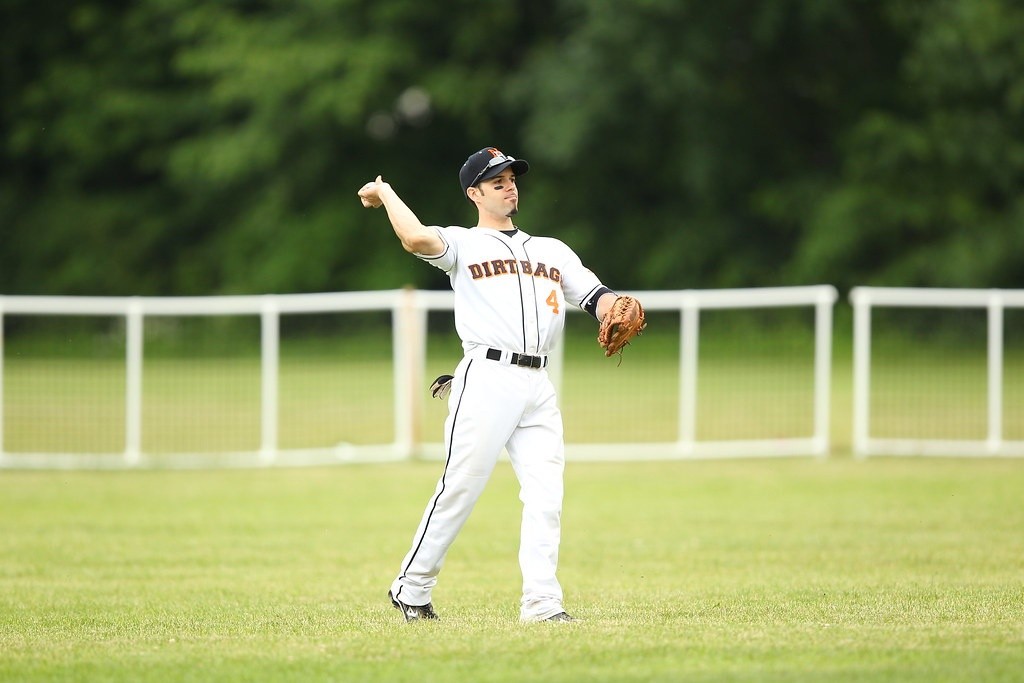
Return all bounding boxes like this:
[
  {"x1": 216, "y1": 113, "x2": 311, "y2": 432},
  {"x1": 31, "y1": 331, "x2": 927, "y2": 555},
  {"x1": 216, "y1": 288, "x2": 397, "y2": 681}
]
[{"x1": 355, "y1": 146, "x2": 632, "y2": 625}]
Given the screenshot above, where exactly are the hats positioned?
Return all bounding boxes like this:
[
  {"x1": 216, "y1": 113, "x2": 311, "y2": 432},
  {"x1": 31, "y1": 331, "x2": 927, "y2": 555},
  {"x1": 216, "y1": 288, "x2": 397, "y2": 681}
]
[{"x1": 458, "y1": 146, "x2": 530, "y2": 199}]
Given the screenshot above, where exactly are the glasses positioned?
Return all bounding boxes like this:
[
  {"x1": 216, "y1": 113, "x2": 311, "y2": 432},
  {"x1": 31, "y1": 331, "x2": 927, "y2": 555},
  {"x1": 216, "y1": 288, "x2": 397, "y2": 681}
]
[{"x1": 469, "y1": 155, "x2": 516, "y2": 187}]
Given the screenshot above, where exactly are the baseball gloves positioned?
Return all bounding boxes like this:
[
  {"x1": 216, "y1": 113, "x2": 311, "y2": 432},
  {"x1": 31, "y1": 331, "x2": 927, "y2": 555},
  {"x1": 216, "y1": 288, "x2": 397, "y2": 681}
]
[{"x1": 596, "y1": 295, "x2": 648, "y2": 358}]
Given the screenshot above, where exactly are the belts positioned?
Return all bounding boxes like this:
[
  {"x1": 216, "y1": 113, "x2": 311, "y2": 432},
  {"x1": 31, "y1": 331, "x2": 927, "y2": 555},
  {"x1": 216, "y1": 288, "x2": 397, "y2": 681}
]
[{"x1": 485, "y1": 348, "x2": 547, "y2": 370}]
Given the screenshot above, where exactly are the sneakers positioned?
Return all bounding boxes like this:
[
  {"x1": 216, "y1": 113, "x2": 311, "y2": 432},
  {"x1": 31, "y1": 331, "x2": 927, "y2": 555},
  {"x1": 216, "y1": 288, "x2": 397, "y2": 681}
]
[
  {"x1": 542, "y1": 611, "x2": 580, "y2": 624},
  {"x1": 388, "y1": 589, "x2": 442, "y2": 624}
]
